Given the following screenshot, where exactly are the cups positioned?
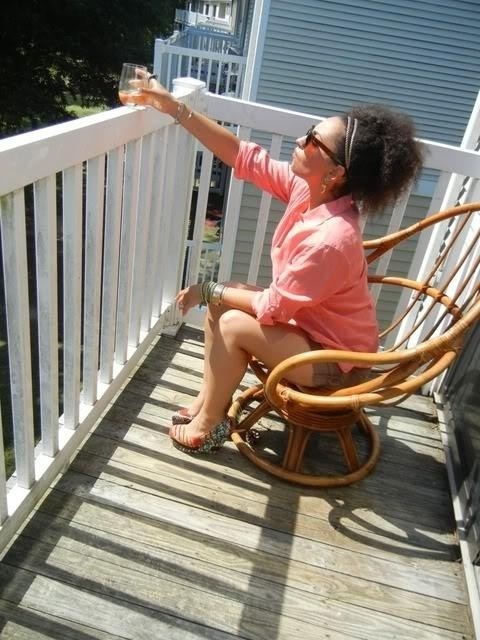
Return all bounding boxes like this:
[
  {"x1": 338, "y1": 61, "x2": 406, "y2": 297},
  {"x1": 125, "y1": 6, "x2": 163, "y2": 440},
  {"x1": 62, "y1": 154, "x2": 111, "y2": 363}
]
[{"x1": 119, "y1": 63, "x2": 148, "y2": 106}]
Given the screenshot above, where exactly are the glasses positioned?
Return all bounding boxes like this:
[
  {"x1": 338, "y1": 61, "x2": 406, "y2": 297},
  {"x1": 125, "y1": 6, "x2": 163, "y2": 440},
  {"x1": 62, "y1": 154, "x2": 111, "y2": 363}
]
[{"x1": 304, "y1": 124, "x2": 345, "y2": 169}]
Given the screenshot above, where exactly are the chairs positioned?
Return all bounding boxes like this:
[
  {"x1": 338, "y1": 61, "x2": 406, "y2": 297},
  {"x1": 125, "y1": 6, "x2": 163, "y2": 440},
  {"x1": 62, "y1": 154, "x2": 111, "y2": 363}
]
[{"x1": 228, "y1": 199, "x2": 480, "y2": 489}]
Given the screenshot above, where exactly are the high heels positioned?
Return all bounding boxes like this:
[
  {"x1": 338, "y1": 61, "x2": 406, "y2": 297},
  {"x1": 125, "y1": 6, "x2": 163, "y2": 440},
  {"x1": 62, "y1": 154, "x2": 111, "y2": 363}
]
[
  {"x1": 169, "y1": 417, "x2": 232, "y2": 454},
  {"x1": 170, "y1": 406, "x2": 196, "y2": 426}
]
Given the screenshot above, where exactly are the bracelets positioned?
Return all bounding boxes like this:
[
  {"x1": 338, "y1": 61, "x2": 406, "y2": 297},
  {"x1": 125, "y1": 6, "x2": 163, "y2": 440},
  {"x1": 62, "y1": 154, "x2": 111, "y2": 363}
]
[
  {"x1": 173, "y1": 102, "x2": 194, "y2": 124},
  {"x1": 198, "y1": 281, "x2": 225, "y2": 310}
]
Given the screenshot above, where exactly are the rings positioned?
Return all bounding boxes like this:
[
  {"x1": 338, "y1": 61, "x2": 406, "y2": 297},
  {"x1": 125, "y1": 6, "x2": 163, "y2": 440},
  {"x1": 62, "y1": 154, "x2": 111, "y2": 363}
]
[
  {"x1": 146, "y1": 74, "x2": 158, "y2": 85},
  {"x1": 179, "y1": 303, "x2": 184, "y2": 309}
]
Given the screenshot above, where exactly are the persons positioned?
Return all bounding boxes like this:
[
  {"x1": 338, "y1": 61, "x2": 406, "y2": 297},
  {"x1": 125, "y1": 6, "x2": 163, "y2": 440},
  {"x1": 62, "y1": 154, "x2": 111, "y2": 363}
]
[{"x1": 117, "y1": 65, "x2": 425, "y2": 457}]
